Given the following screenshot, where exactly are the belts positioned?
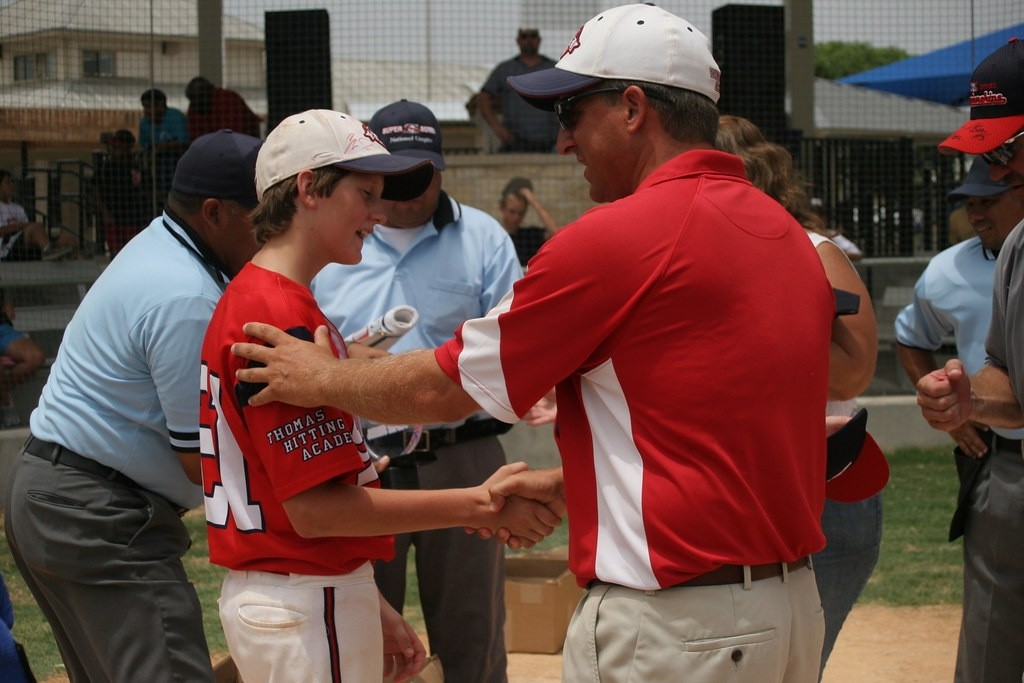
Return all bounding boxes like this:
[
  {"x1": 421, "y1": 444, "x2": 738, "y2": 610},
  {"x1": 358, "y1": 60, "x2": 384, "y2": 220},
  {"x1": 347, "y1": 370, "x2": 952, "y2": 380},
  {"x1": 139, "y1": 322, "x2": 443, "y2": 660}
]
[
  {"x1": 25, "y1": 431, "x2": 145, "y2": 490},
  {"x1": 985, "y1": 433, "x2": 1021, "y2": 453},
  {"x1": 674, "y1": 556, "x2": 810, "y2": 586},
  {"x1": 362, "y1": 419, "x2": 514, "y2": 457}
]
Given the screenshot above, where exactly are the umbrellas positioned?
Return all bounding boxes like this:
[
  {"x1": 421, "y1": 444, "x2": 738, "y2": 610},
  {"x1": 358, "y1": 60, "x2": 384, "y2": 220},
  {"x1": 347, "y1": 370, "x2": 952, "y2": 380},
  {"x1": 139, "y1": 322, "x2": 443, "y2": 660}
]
[{"x1": 834, "y1": 21, "x2": 1024, "y2": 107}]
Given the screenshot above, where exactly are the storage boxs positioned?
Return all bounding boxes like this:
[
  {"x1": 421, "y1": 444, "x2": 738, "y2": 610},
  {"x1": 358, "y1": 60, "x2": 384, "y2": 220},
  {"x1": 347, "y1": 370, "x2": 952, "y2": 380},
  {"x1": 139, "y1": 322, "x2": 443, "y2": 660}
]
[
  {"x1": 502, "y1": 558, "x2": 586, "y2": 654},
  {"x1": 212, "y1": 653, "x2": 444, "y2": 683}
]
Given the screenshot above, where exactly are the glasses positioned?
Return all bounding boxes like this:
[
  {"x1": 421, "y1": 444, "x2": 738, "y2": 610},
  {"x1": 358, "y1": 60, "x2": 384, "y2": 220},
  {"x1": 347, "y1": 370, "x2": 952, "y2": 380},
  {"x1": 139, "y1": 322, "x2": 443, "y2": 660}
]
[
  {"x1": 980, "y1": 131, "x2": 1024, "y2": 167},
  {"x1": 552, "y1": 86, "x2": 667, "y2": 130}
]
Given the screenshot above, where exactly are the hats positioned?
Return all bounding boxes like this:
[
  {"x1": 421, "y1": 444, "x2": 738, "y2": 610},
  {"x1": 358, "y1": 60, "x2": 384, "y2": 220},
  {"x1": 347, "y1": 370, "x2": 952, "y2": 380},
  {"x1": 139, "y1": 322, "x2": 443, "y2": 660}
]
[
  {"x1": 507, "y1": 3, "x2": 722, "y2": 112},
  {"x1": 254, "y1": 109, "x2": 435, "y2": 201},
  {"x1": 946, "y1": 156, "x2": 1020, "y2": 205},
  {"x1": 108, "y1": 129, "x2": 136, "y2": 146},
  {"x1": 367, "y1": 98, "x2": 446, "y2": 170},
  {"x1": 171, "y1": 129, "x2": 263, "y2": 203},
  {"x1": 937, "y1": 35, "x2": 1024, "y2": 155}
]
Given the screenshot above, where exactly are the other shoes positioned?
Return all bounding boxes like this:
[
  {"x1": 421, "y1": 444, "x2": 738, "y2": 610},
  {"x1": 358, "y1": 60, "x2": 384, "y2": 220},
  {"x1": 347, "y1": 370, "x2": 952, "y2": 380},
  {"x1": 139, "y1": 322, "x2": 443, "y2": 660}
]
[{"x1": 0, "y1": 391, "x2": 20, "y2": 427}]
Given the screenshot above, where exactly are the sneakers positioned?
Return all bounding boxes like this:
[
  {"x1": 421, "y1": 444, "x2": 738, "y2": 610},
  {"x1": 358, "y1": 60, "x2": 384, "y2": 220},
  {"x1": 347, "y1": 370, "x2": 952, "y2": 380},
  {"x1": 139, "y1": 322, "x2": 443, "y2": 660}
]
[{"x1": 42, "y1": 242, "x2": 73, "y2": 260}]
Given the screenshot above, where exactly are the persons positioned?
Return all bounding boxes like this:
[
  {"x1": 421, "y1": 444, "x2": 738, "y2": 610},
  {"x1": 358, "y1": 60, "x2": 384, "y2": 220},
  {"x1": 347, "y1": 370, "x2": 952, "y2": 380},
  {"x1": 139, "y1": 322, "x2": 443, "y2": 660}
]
[
  {"x1": 465, "y1": 28, "x2": 559, "y2": 155},
  {"x1": 0, "y1": 75, "x2": 262, "y2": 263},
  {"x1": 309, "y1": 97, "x2": 524, "y2": 683},
  {"x1": 917, "y1": 37, "x2": 1024, "y2": 683},
  {"x1": 198, "y1": 110, "x2": 563, "y2": 683},
  {"x1": 232, "y1": 4, "x2": 835, "y2": 683},
  {"x1": 894, "y1": 158, "x2": 1024, "y2": 542},
  {"x1": 7, "y1": 126, "x2": 264, "y2": 683},
  {"x1": 501, "y1": 177, "x2": 557, "y2": 268},
  {"x1": 521, "y1": 117, "x2": 889, "y2": 683},
  {"x1": 0, "y1": 289, "x2": 45, "y2": 683},
  {"x1": 811, "y1": 198, "x2": 865, "y2": 261}
]
